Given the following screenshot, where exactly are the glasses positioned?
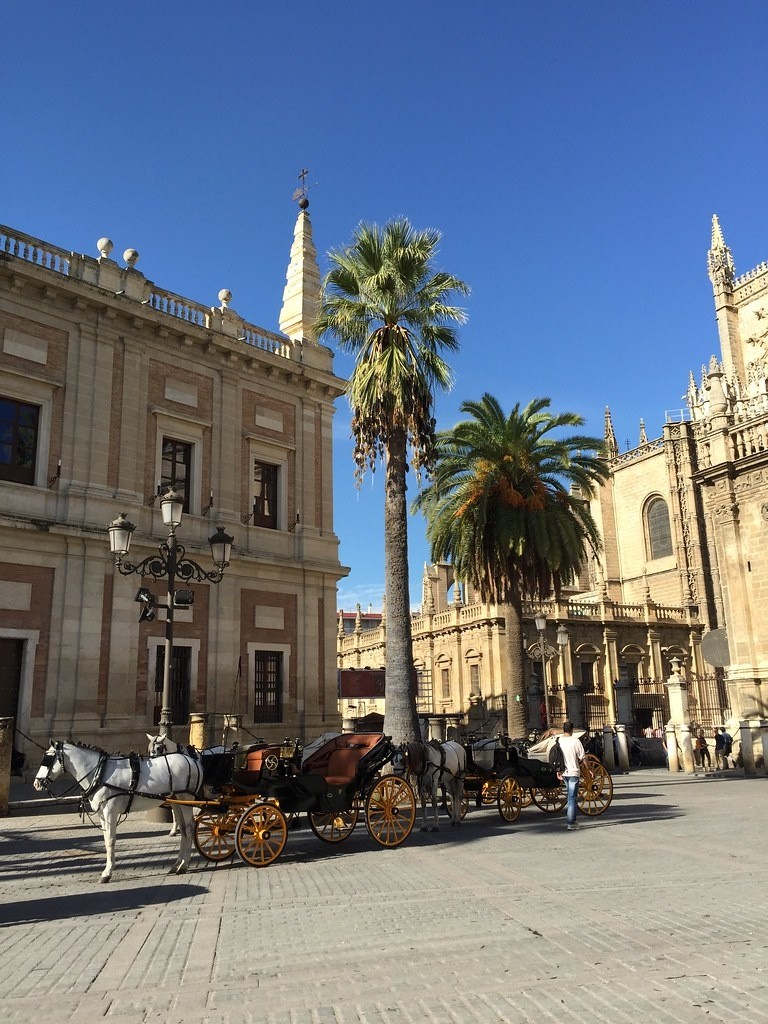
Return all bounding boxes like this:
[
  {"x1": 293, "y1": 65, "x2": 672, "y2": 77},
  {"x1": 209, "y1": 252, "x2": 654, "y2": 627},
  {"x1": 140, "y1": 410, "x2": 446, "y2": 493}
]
[{"x1": 571, "y1": 728, "x2": 575, "y2": 731}]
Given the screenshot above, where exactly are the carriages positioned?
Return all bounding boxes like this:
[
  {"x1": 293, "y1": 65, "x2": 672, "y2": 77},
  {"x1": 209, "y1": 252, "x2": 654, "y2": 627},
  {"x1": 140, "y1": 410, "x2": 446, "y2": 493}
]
[
  {"x1": 33, "y1": 728, "x2": 417, "y2": 883},
  {"x1": 390, "y1": 727, "x2": 613, "y2": 833}
]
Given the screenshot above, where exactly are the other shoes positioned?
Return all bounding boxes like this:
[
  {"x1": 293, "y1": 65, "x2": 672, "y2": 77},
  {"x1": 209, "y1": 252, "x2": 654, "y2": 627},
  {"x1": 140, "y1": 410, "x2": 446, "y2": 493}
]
[
  {"x1": 708, "y1": 767, "x2": 714, "y2": 772},
  {"x1": 702, "y1": 767, "x2": 707, "y2": 772}
]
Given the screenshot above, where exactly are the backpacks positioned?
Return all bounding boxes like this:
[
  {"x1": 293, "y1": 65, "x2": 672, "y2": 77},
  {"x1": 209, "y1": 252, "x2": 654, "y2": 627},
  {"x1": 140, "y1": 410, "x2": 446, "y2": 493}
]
[
  {"x1": 548, "y1": 737, "x2": 565, "y2": 773},
  {"x1": 696, "y1": 739, "x2": 703, "y2": 750}
]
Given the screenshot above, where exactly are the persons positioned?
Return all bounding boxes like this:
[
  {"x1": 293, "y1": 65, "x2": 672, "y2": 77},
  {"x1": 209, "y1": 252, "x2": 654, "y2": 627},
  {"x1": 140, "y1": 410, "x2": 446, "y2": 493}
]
[
  {"x1": 636, "y1": 725, "x2": 663, "y2": 739},
  {"x1": 662, "y1": 727, "x2": 683, "y2": 771},
  {"x1": 432, "y1": 734, "x2": 489, "y2": 745},
  {"x1": 501, "y1": 731, "x2": 511, "y2": 748},
  {"x1": 698, "y1": 730, "x2": 714, "y2": 772},
  {"x1": 714, "y1": 727, "x2": 737, "y2": 770},
  {"x1": 528, "y1": 732, "x2": 536, "y2": 742},
  {"x1": 548, "y1": 722, "x2": 590, "y2": 830}
]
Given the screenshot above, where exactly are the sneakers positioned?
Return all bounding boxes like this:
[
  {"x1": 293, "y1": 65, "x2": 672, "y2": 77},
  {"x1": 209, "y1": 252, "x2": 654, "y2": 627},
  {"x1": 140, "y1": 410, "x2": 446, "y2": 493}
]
[{"x1": 567, "y1": 823, "x2": 582, "y2": 830}]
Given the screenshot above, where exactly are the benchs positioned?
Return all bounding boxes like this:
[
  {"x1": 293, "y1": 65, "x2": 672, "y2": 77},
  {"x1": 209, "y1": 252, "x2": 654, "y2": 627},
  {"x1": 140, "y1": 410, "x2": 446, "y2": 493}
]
[{"x1": 325, "y1": 748, "x2": 371, "y2": 783}]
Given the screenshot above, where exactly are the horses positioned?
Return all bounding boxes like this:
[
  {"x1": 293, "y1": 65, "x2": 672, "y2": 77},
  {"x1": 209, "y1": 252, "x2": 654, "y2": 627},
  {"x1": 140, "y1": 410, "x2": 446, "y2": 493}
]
[
  {"x1": 390, "y1": 739, "x2": 467, "y2": 831},
  {"x1": 145, "y1": 733, "x2": 229, "y2": 837},
  {"x1": 33, "y1": 739, "x2": 203, "y2": 883}
]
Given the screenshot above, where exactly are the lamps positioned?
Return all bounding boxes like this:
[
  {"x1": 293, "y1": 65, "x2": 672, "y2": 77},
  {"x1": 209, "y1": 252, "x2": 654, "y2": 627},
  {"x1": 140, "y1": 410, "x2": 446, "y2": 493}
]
[
  {"x1": 203, "y1": 489, "x2": 214, "y2": 516},
  {"x1": 49, "y1": 459, "x2": 62, "y2": 489},
  {"x1": 288, "y1": 508, "x2": 300, "y2": 533},
  {"x1": 240, "y1": 498, "x2": 257, "y2": 524},
  {"x1": 148, "y1": 479, "x2": 162, "y2": 507}
]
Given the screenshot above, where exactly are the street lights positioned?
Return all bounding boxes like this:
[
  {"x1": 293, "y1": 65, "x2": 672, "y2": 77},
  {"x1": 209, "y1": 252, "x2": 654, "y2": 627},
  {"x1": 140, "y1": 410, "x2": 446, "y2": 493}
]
[
  {"x1": 107, "y1": 486, "x2": 234, "y2": 741},
  {"x1": 523, "y1": 609, "x2": 569, "y2": 728}
]
